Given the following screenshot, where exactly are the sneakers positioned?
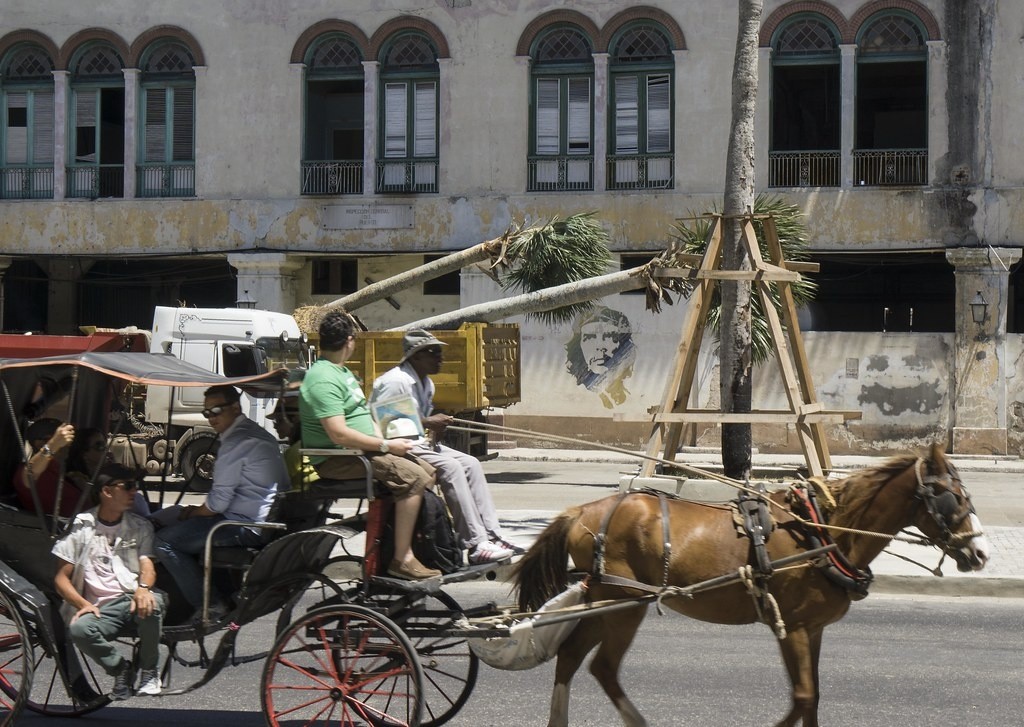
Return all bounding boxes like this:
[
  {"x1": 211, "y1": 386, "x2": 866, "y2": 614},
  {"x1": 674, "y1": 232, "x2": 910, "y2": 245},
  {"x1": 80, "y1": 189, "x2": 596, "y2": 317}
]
[
  {"x1": 387, "y1": 558, "x2": 441, "y2": 581},
  {"x1": 467, "y1": 538, "x2": 527, "y2": 564},
  {"x1": 136, "y1": 667, "x2": 162, "y2": 696},
  {"x1": 111, "y1": 660, "x2": 137, "y2": 700}
]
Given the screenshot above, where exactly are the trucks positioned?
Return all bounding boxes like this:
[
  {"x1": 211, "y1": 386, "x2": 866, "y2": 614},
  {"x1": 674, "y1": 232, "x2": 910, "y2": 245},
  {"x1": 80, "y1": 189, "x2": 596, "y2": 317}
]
[{"x1": 111, "y1": 306, "x2": 317, "y2": 492}]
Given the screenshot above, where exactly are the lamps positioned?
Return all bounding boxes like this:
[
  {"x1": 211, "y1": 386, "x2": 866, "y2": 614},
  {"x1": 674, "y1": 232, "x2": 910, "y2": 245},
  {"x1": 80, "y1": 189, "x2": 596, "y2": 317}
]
[{"x1": 969, "y1": 290, "x2": 988, "y2": 342}]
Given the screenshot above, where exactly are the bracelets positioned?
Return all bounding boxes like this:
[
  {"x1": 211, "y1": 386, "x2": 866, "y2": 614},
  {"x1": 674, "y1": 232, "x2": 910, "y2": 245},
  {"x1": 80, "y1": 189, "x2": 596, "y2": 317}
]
[{"x1": 42, "y1": 444, "x2": 56, "y2": 459}]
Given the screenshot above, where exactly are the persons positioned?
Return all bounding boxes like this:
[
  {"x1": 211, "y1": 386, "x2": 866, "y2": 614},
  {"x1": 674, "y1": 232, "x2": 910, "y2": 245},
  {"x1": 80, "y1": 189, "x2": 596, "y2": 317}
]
[
  {"x1": 368, "y1": 328, "x2": 529, "y2": 565},
  {"x1": 51, "y1": 463, "x2": 162, "y2": 701},
  {"x1": 298, "y1": 312, "x2": 442, "y2": 580},
  {"x1": 14, "y1": 418, "x2": 98, "y2": 519},
  {"x1": 153, "y1": 385, "x2": 290, "y2": 624},
  {"x1": 64, "y1": 427, "x2": 152, "y2": 516}
]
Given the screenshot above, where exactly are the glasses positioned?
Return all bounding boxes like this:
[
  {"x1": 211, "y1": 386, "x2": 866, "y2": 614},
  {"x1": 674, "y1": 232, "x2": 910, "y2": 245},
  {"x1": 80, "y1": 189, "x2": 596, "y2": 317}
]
[
  {"x1": 111, "y1": 481, "x2": 139, "y2": 491},
  {"x1": 417, "y1": 346, "x2": 442, "y2": 354},
  {"x1": 90, "y1": 441, "x2": 107, "y2": 451},
  {"x1": 202, "y1": 402, "x2": 234, "y2": 418}
]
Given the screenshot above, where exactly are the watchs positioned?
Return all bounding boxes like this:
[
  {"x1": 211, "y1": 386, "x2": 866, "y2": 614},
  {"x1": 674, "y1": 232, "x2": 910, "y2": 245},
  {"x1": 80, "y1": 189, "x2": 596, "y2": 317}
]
[
  {"x1": 380, "y1": 440, "x2": 389, "y2": 453},
  {"x1": 139, "y1": 583, "x2": 150, "y2": 589}
]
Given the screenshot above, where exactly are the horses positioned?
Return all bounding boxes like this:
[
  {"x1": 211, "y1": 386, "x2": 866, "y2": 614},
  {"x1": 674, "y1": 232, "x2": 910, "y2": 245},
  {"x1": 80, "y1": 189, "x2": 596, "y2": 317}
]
[{"x1": 502, "y1": 443, "x2": 991, "y2": 727}]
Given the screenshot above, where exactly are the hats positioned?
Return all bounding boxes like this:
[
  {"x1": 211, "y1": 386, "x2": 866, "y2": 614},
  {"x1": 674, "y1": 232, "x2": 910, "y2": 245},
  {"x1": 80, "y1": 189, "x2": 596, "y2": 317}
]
[
  {"x1": 95, "y1": 463, "x2": 146, "y2": 490},
  {"x1": 399, "y1": 329, "x2": 449, "y2": 364}
]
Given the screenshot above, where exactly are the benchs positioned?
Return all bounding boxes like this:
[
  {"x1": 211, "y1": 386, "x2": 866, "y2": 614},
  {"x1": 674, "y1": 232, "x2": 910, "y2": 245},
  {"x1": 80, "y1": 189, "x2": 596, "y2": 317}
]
[
  {"x1": 286, "y1": 395, "x2": 395, "y2": 503},
  {"x1": 196, "y1": 493, "x2": 334, "y2": 581}
]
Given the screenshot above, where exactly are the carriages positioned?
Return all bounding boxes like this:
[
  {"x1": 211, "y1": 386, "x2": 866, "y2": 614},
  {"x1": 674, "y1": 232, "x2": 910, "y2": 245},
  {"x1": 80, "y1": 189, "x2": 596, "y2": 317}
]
[{"x1": 0, "y1": 333, "x2": 990, "y2": 727}]
